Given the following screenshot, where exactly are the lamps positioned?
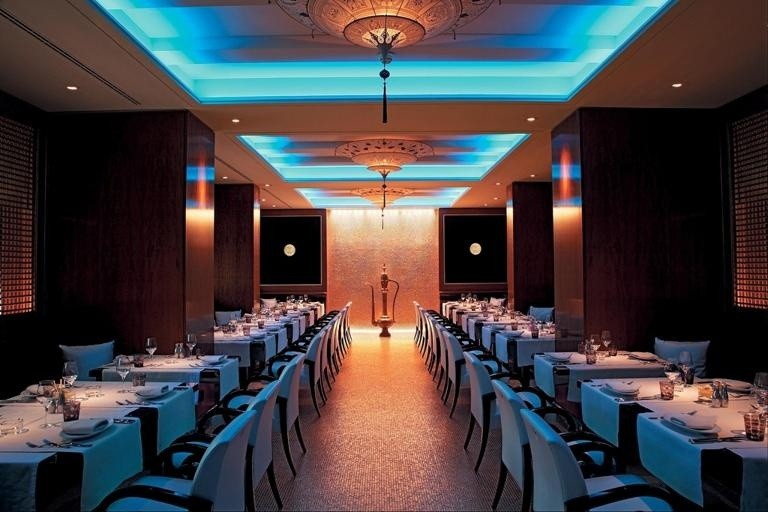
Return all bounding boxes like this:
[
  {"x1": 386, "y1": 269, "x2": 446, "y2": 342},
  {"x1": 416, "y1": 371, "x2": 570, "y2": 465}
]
[
  {"x1": 352, "y1": 187, "x2": 413, "y2": 210},
  {"x1": 275, "y1": 1, "x2": 502, "y2": 50},
  {"x1": 334, "y1": 140, "x2": 434, "y2": 175}
]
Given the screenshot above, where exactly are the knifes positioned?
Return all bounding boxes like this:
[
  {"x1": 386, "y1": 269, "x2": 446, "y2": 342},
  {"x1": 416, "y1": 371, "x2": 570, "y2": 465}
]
[
  {"x1": 689, "y1": 436, "x2": 748, "y2": 442},
  {"x1": 551, "y1": 362, "x2": 583, "y2": 365}
]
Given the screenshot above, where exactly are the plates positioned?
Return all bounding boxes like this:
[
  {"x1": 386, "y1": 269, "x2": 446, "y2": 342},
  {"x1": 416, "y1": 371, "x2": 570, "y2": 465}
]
[
  {"x1": 58, "y1": 416, "x2": 114, "y2": 439},
  {"x1": 712, "y1": 379, "x2": 754, "y2": 393},
  {"x1": 544, "y1": 352, "x2": 573, "y2": 360},
  {"x1": 200, "y1": 355, "x2": 229, "y2": 365},
  {"x1": 476, "y1": 317, "x2": 488, "y2": 321},
  {"x1": 135, "y1": 388, "x2": 174, "y2": 400},
  {"x1": 467, "y1": 312, "x2": 479, "y2": 316},
  {"x1": 629, "y1": 351, "x2": 657, "y2": 361},
  {"x1": 605, "y1": 380, "x2": 640, "y2": 395},
  {"x1": 492, "y1": 325, "x2": 505, "y2": 330},
  {"x1": 662, "y1": 413, "x2": 721, "y2": 434}
]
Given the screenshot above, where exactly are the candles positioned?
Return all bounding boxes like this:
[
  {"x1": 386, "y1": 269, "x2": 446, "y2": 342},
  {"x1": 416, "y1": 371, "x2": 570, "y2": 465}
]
[{"x1": 441, "y1": 302, "x2": 447, "y2": 316}]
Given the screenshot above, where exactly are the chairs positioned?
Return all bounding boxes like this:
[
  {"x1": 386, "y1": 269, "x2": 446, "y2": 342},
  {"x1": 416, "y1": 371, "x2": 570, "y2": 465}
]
[
  {"x1": 490, "y1": 379, "x2": 618, "y2": 512},
  {"x1": 442, "y1": 330, "x2": 501, "y2": 418},
  {"x1": 435, "y1": 323, "x2": 485, "y2": 392},
  {"x1": 100, "y1": 409, "x2": 255, "y2": 512},
  {"x1": 518, "y1": 407, "x2": 681, "y2": 511},
  {"x1": 296, "y1": 316, "x2": 339, "y2": 382},
  {"x1": 337, "y1": 301, "x2": 353, "y2": 366},
  {"x1": 422, "y1": 313, "x2": 475, "y2": 364},
  {"x1": 416, "y1": 307, "x2": 454, "y2": 347},
  {"x1": 257, "y1": 331, "x2": 326, "y2": 417},
  {"x1": 292, "y1": 324, "x2": 333, "y2": 401},
  {"x1": 429, "y1": 319, "x2": 479, "y2": 380},
  {"x1": 412, "y1": 302, "x2": 441, "y2": 340},
  {"x1": 166, "y1": 380, "x2": 283, "y2": 512},
  {"x1": 414, "y1": 303, "x2": 448, "y2": 344},
  {"x1": 200, "y1": 354, "x2": 307, "y2": 479},
  {"x1": 263, "y1": 306, "x2": 314, "y2": 328},
  {"x1": 324, "y1": 310, "x2": 341, "y2": 317},
  {"x1": 460, "y1": 350, "x2": 557, "y2": 473}
]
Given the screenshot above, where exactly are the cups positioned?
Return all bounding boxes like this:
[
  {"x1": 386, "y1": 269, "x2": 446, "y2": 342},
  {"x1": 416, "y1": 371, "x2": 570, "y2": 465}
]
[
  {"x1": 510, "y1": 312, "x2": 515, "y2": 319},
  {"x1": 664, "y1": 358, "x2": 682, "y2": 384},
  {"x1": 467, "y1": 292, "x2": 472, "y2": 298},
  {"x1": 299, "y1": 296, "x2": 304, "y2": 305},
  {"x1": 515, "y1": 311, "x2": 520, "y2": 315},
  {"x1": 608, "y1": 344, "x2": 617, "y2": 356},
  {"x1": 115, "y1": 355, "x2": 131, "y2": 394},
  {"x1": 458, "y1": 299, "x2": 462, "y2": 305},
  {"x1": 245, "y1": 315, "x2": 252, "y2": 323},
  {"x1": 175, "y1": 342, "x2": 183, "y2": 361},
  {"x1": 258, "y1": 321, "x2": 264, "y2": 329},
  {"x1": 133, "y1": 353, "x2": 145, "y2": 367},
  {"x1": 531, "y1": 329, "x2": 539, "y2": 339},
  {"x1": 290, "y1": 295, "x2": 295, "y2": 303},
  {"x1": 35, "y1": 380, "x2": 59, "y2": 430},
  {"x1": 473, "y1": 294, "x2": 477, "y2": 301},
  {"x1": 461, "y1": 293, "x2": 466, "y2": 303},
  {"x1": 221, "y1": 324, "x2": 230, "y2": 337},
  {"x1": 590, "y1": 334, "x2": 601, "y2": 353},
  {"x1": 274, "y1": 311, "x2": 280, "y2": 321},
  {"x1": 242, "y1": 324, "x2": 251, "y2": 336},
  {"x1": 483, "y1": 310, "x2": 488, "y2": 317},
  {"x1": 493, "y1": 314, "x2": 499, "y2": 322},
  {"x1": 186, "y1": 333, "x2": 198, "y2": 360},
  {"x1": 511, "y1": 322, "x2": 518, "y2": 330},
  {"x1": 679, "y1": 351, "x2": 692, "y2": 369},
  {"x1": 132, "y1": 371, "x2": 146, "y2": 388},
  {"x1": 586, "y1": 349, "x2": 596, "y2": 365},
  {"x1": 62, "y1": 400, "x2": 80, "y2": 422},
  {"x1": 281, "y1": 308, "x2": 288, "y2": 316},
  {"x1": 145, "y1": 338, "x2": 157, "y2": 368},
  {"x1": 483, "y1": 298, "x2": 488, "y2": 303},
  {"x1": 286, "y1": 296, "x2": 291, "y2": 306},
  {"x1": 293, "y1": 305, "x2": 299, "y2": 312},
  {"x1": 682, "y1": 367, "x2": 695, "y2": 385},
  {"x1": 744, "y1": 412, "x2": 766, "y2": 441},
  {"x1": 659, "y1": 380, "x2": 675, "y2": 400},
  {"x1": 304, "y1": 293, "x2": 308, "y2": 303},
  {"x1": 61, "y1": 361, "x2": 79, "y2": 391},
  {"x1": 600, "y1": 330, "x2": 612, "y2": 358},
  {"x1": 471, "y1": 305, "x2": 476, "y2": 312},
  {"x1": 754, "y1": 373, "x2": 768, "y2": 396}
]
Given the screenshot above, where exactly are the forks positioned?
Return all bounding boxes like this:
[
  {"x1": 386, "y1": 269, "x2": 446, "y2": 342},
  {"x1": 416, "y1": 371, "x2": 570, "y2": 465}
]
[
  {"x1": 648, "y1": 410, "x2": 699, "y2": 421},
  {"x1": 729, "y1": 393, "x2": 754, "y2": 399},
  {"x1": 125, "y1": 399, "x2": 165, "y2": 406},
  {"x1": 116, "y1": 400, "x2": 150, "y2": 406},
  {"x1": 25, "y1": 441, "x2": 71, "y2": 450}
]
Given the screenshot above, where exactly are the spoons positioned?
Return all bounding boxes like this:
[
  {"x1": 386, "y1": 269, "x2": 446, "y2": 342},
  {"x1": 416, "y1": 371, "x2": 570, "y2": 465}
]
[{"x1": 41, "y1": 438, "x2": 93, "y2": 448}]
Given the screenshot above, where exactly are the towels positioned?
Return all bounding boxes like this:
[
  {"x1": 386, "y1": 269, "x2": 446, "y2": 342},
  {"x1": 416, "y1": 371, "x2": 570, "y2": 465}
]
[
  {"x1": 199, "y1": 355, "x2": 225, "y2": 365},
  {"x1": 267, "y1": 323, "x2": 280, "y2": 331},
  {"x1": 299, "y1": 307, "x2": 308, "y2": 312},
  {"x1": 607, "y1": 377, "x2": 641, "y2": 395},
  {"x1": 64, "y1": 418, "x2": 108, "y2": 434},
  {"x1": 136, "y1": 384, "x2": 169, "y2": 398},
  {"x1": 677, "y1": 413, "x2": 718, "y2": 428},
  {"x1": 287, "y1": 310, "x2": 299, "y2": 317},
  {"x1": 545, "y1": 351, "x2": 573, "y2": 362},
  {"x1": 714, "y1": 377, "x2": 753, "y2": 389},
  {"x1": 23, "y1": 384, "x2": 44, "y2": 395},
  {"x1": 278, "y1": 314, "x2": 291, "y2": 324},
  {"x1": 629, "y1": 352, "x2": 657, "y2": 360},
  {"x1": 249, "y1": 329, "x2": 267, "y2": 338}
]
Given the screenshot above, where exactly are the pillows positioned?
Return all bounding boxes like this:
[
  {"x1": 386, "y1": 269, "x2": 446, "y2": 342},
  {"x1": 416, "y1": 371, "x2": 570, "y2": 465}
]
[
  {"x1": 653, "y1": 336, "x2": 709, "y2": 379},
  {"x1": 530, "y1": 306, "x2": 555, "y2": 322},
  {"x1": 261, "y1": 298, "x2": 275, "y2": 306},
  {"x1": 60, "y1": 340, "x2": 115, "y2": 381},
  {"x1": 214, "y1": 310, "x2": 241, "y2": 325}
]
